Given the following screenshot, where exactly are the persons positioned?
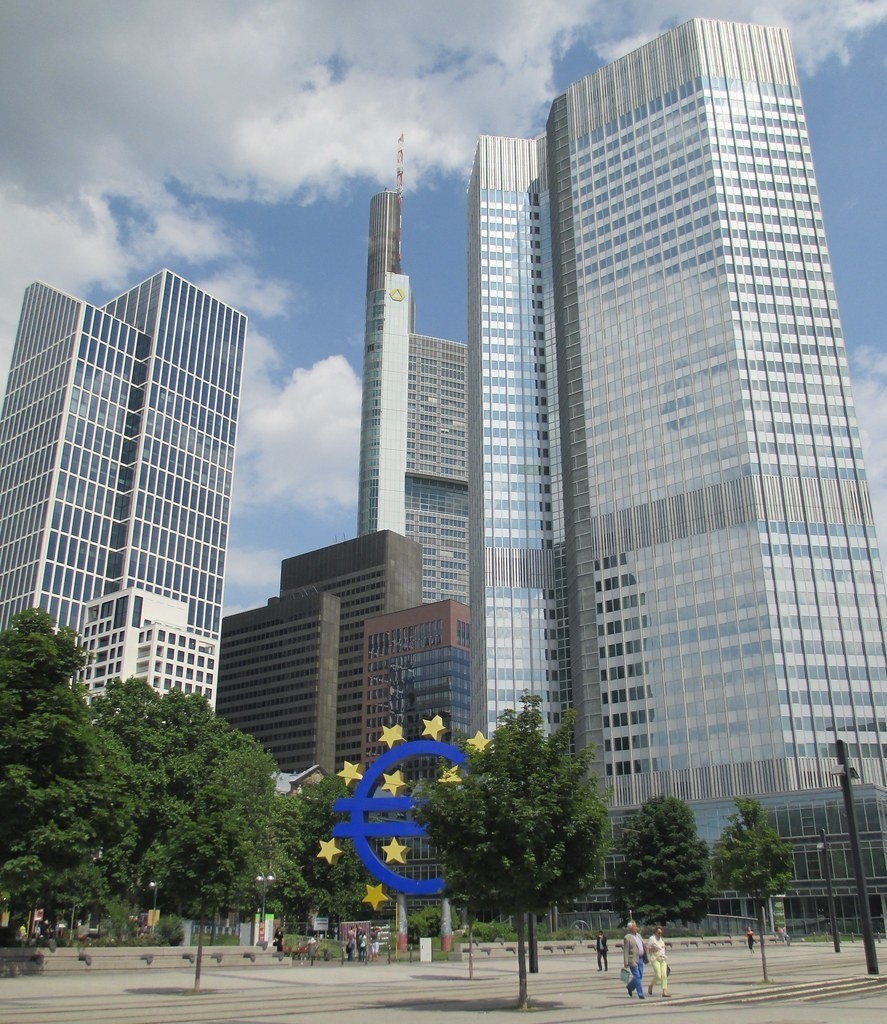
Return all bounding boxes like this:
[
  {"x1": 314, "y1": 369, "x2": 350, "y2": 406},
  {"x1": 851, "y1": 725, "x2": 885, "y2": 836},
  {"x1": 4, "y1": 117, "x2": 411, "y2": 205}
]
[
  {"x1": 18, "y1": 923, "x2": 26, "y2": 941},
  {"x1": 624, "y1": 922, "x2": 672, "y2": 1000},
  {"x1": 272, "y1": 926, "x2": 284, "y2": 952},
  {"x1": 746, "y1": 926, "x2": 757, "y2": 955},
  {"x1": 347, "y1": 924, "x2": 379, "y2": 963},
  {"x1": 596, "y1": 930, "x2": 608, "y2": 972}
]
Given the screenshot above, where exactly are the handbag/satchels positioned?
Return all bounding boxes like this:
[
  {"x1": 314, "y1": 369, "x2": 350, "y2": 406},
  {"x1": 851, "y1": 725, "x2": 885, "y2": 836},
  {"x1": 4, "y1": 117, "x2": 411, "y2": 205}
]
[{"x1": 620, "y1": 966, "x2": 628, "y2": 983}]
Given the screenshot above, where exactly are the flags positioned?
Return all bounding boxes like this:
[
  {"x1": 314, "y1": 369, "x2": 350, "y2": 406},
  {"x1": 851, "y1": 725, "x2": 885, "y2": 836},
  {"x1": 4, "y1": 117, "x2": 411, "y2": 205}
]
[{"x1": 147, "y1": 909, "x2": 160, "y2": 926}]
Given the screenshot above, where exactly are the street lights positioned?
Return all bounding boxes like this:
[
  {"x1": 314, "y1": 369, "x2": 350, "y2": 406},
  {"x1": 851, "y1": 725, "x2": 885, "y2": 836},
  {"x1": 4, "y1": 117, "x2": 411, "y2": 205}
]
[
  {"x1": 147, "y1": 877, "x2": 165, "y2": 943},
  {"x1": 828, "y1": 738, "x2": 880, "y2": 980},
  {"x1": 256, "y1": 867, "x2": 276, "y2": 949},
  {"x1": 816, "y1": 826, "x2": 841, "y2": 955}
]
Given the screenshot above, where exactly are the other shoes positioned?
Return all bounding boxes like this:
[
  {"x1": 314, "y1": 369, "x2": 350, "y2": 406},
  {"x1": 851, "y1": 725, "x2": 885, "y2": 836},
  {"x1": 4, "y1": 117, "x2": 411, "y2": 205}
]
[
  {"x1": 648, "y1": 985, "x2": 654, "y2": 995},
  {"x1": 640, "y1": 996, "x2": 644, "y2": 998},
  {"x1": 660, "y1": 994, "x2": 671, "y2": 997},
  {"x1": 627, "y1": 986, "x2": 633, "y2": 997}
]
